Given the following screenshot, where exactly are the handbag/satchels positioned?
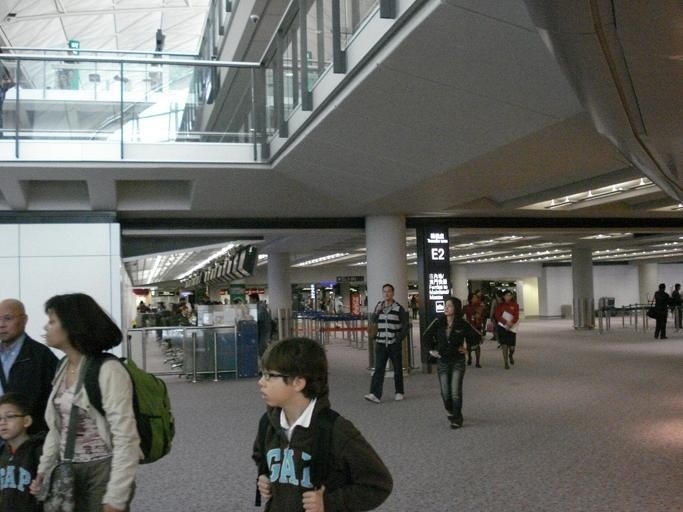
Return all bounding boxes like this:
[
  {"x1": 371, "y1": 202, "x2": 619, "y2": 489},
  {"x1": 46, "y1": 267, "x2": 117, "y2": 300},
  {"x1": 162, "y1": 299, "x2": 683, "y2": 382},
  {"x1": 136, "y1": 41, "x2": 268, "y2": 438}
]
[
  {"x1": 646, "y1": 307, "x2": 657, "y2": 319},
  {"x1": 365, "y1": 321, "x2": 378, "y2": 340}
]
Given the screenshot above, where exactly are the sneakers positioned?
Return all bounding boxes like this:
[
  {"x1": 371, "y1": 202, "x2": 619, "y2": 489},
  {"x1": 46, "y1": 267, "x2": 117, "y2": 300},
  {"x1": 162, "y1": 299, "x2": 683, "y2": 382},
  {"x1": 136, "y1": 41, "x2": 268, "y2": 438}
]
[
  {"x1": 504, "y1": 363, "x2": 509, "y2": 370},
  {"x1": 659, "y1": 336, "x2": 668, "y2": 339},
  {"x1": 509, "y1": 356, "x2": 515, "y2": 365},
  {"x1": 467, "y1": 357, "x2": 472, "y2": 365},
  {"x1": 475, "y1": 364, "x2": 481, "y2": 368},
  {"x1": 446, "y1": 412, "x2": 463, "y2": 430},
  {"x1": 393, "y1": 392, "x2": 404, "y2": 401},
  {"x1": 654, "y1": 335, "x2": 658, "y2": 339},
  {"x1": 363, "y1": 393, "x2": 383, "y2": 403}
]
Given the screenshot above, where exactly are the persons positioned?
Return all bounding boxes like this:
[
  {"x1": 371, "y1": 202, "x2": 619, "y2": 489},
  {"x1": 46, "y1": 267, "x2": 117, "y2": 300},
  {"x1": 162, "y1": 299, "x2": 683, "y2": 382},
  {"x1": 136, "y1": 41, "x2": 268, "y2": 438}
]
[
  {"x1": 463, "y1": 282, "x2": 520, "y2": 369},
  {"x1": 137, "y1": 290, "x2": 273, "y2": 373},
  {"x1": 654, "y1": 283, "x2": 669, "y2": 339},
  {"x1": 672, "y1": 283, "x2": 683, "y2": 330},
  {"x1": 411, "y1": 297, "x2": 417, "y2": 320},
  {"x1": 306, "y1": 294, "x2": 343, "y2": 313},
  {"x1": 31, "y1": 294, "x2": 142, "y2": 511},
  {"x1": 365, "y1": 284, "x2": 408, "y2": 402},
  {"x1": 0, "y1": 298, "x2": 59, "y2": 434},
  {"x1": 421, "y1": 296, "x2": 478, "y2": 428},
  {"x1": 1, "y1": 393, "x2": 45, "y2": 511},
  {"x1": 250, "y1": 338, "x2": 392, "y2": 511}
]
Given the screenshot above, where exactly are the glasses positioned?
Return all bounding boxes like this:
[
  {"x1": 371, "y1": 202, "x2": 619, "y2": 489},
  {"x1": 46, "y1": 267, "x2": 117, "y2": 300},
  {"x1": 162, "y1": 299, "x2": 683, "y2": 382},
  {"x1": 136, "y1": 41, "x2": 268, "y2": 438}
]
[
  {"x1": 0, "y1": 413, "x2": 26, "y2": 420},
  {"x1": 257, "y1": 370, "x2": 290, "y2": 380}
]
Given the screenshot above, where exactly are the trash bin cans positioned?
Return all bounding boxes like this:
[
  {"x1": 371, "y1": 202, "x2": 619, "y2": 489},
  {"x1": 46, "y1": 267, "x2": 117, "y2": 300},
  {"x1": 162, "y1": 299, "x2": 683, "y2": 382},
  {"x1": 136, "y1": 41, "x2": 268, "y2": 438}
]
[{"x1": 560, "y1": 304, "x2": 571, "y2": 320}]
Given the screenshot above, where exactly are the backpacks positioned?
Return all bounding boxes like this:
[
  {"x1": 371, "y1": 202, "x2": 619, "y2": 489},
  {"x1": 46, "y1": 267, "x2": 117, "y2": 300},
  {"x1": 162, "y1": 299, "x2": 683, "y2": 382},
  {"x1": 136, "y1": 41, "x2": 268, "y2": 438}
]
[{"x1": 81, "y1": 350, "x2": 175, "y2": 466}]
